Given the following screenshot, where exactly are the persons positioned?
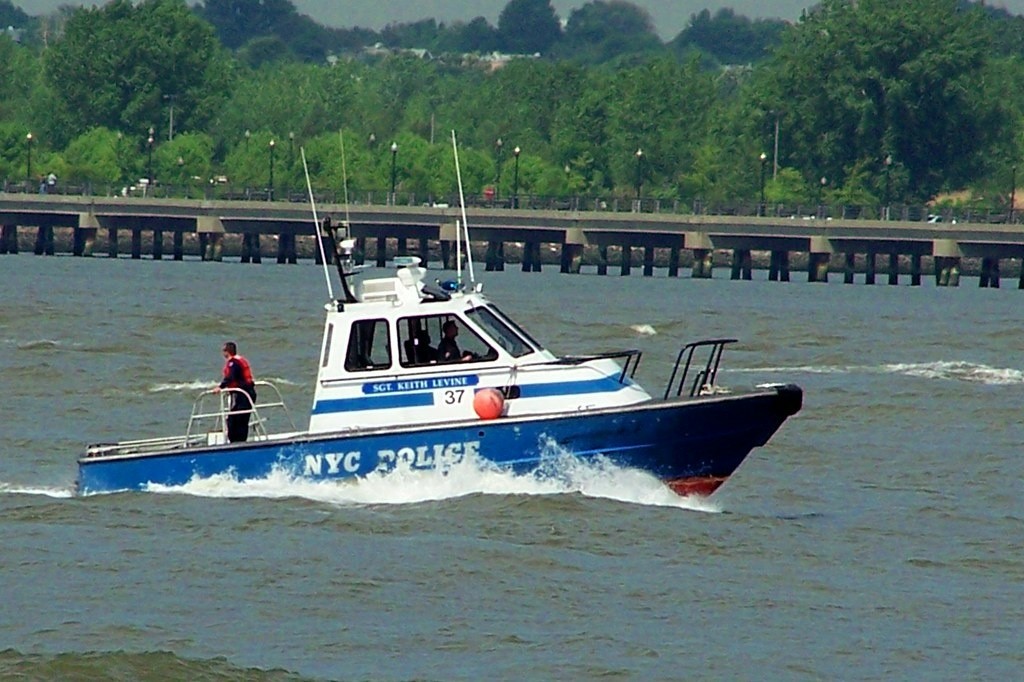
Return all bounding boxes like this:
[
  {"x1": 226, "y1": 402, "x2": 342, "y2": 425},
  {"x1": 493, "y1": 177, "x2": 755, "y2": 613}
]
[
  {"x1": 213, "y1": 342, "x2": 256, "y2": 443},
  {"x1": 417, "y1": 330, "x2": 447, "y2": 362},
  {"x1": 38, "y1": 171, "x2": 56, "y2": 194},
  {"x1": 439, "y1": 321, "x2": 472, "y2": 361}
]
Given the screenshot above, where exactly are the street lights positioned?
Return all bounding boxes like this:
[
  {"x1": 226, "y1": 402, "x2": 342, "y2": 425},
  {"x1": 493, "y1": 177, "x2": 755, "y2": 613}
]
[
  {"x1": 1008, "y1": 165, "x2": 1016, "y2": 224},
  {"x1": 147, "y1": 128, "x2": 154, "y2": 186},
  {"x1": 633, "y1": 148, "x2": 642, "y2": 212},
  {"x1": 881, "y1": 154, "x2": 893, "y2": 220},
  {"x1": 510, "y1": 145, "x2": 519, "y2": 209},
  {"x1": 757, "y1": 151, "x2": 767, "y2": 217},
  {"x1": 389, "y1": 142, "x2": 398, "y2": 206},
  {"x1": 26, "y1": 132, "x2": 32, "y2": 192},
  {"x1": 289, "y1": 131, "x2": 295, "y2": 164},
  {"x1": 266, "y1": 138, "x2": 275, "y2": 202},
  {"x1": 494, "y1": 138, "x2": 502, "y2": 201}
]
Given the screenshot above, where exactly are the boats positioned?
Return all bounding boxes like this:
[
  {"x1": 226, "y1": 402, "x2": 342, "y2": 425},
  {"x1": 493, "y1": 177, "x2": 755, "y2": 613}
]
[{"x1": 75, "y1": 127, "x2": 805, "y2": 510}]
